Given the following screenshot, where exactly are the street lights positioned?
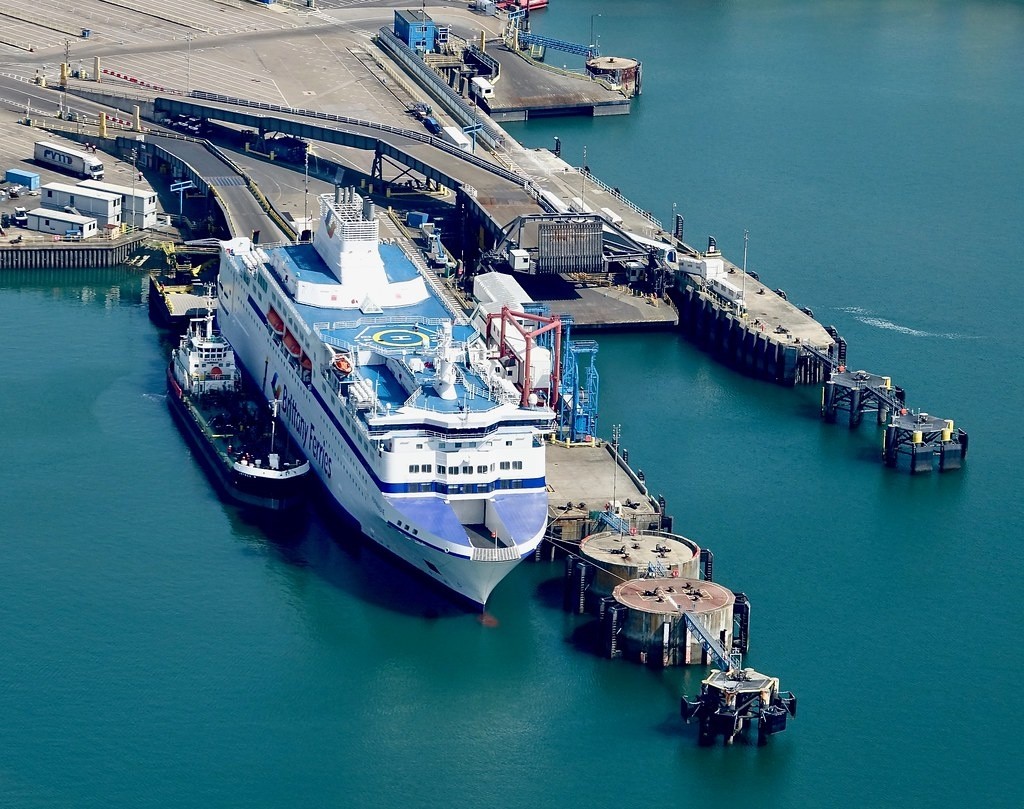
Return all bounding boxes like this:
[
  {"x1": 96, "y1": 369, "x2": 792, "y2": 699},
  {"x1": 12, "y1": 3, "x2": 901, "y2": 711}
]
[
  {"x1": 130, "y1": 147, "x2": 139, "y2": 232},
  {"x1": 185, "y1": 33, "x2": 194, "y2": 92},
  {"x1": 63, "y1": 37, "x2": 72, "y2": 111},
  {"x1": 590, "y1": 14, "x2": 601, "y2": 47}
]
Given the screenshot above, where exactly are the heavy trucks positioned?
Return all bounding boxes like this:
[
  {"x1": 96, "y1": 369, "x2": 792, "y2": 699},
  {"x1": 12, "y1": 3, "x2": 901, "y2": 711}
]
[{"x1": 33, "y1": 141, "x2": 105, "y2": 181}]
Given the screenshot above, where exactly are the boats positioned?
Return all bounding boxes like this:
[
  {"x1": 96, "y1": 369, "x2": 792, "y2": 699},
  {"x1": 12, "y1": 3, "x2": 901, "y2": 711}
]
[
  {"x1": 202, "y1": 145, "x2": 560, "y2": 615},
  {"x1": 168, "y1": 283, "x2": 313, "y2": 519}
]
